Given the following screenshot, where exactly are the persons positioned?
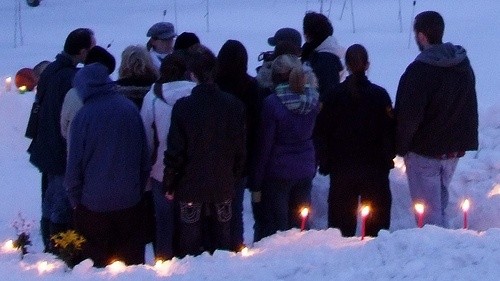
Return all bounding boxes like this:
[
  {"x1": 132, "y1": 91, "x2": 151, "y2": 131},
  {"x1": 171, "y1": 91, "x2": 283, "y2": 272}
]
[
  {"x1": 395, "y1": 10, "x2": 479, "y2": 229},
  {"x1": 26, "y1": 11, "x2": 393, "y2": 266}
]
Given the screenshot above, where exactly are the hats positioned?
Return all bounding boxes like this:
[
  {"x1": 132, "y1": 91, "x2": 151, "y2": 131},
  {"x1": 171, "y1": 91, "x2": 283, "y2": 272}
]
[
  {"x1": 146, "y1": 21, "x2": 177, "y2": 40},
  {"x1": 267, "y1": 27, "x2": 302, "y2": 51},
  {"x1": 173, "y1": 32, "x2": 200, "y2": 51},
  {"x1": 84, "y1": 46, "x2": 116, "y2": 75}
]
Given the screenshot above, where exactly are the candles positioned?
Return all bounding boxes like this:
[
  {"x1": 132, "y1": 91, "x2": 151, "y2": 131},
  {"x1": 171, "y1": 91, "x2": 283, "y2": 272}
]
[
  {"x1": 300, "y1": 208, "x2": 309, "y2": 231},
  {"x1": 414, "y1": 203, "x2": 426, "y2": 228},
  {"x1": 461, "y1": 199, "x2": 470, "y2": 229},
  {"x1": 5, "y1": 76, "x2": 12, "y2": 91},
  {"x1": 359, "y1": 205, "x2": 370, "y2": 239}
]
